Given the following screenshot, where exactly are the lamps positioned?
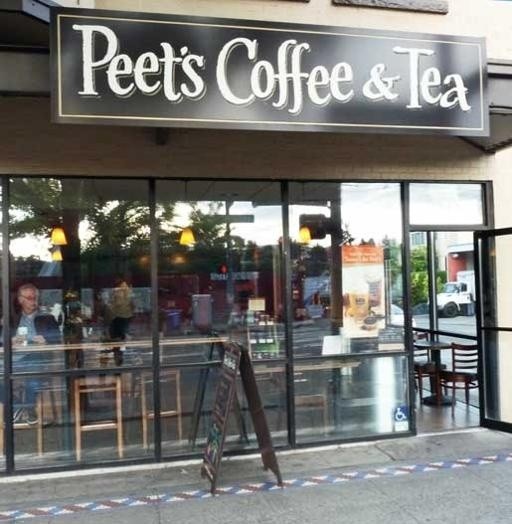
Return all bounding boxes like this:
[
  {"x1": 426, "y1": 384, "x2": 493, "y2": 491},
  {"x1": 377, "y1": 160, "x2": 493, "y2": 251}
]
[{"x1": 49, "y1": 179, "x2": 312, "y2": 247}]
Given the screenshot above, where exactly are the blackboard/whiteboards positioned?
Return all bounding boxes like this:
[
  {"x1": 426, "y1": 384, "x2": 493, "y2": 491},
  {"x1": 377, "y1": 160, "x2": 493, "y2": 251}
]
[{"x1": 203, "y1": 342, "x2": 239, "y2": 480}]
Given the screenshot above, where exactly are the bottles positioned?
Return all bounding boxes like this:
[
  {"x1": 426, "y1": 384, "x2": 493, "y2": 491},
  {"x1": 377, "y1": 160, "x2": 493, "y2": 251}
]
[{"x1": 228, "y1": 311, "x2": 274, "y2": 344}]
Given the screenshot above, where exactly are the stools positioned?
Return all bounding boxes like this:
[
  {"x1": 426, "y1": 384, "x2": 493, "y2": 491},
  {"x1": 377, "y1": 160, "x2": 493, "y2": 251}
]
[{"x1": 3, "y1": 366, "x2": 183, "y2": 466}]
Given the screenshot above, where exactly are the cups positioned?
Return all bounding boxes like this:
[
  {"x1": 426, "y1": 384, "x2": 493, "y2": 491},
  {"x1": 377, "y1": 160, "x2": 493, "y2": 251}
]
[
  {"x1": 17, "y1": 326, "x2": 28, "y2": 347},
  {"x1": 349, "y1": 279, "x2": 382, "y2": 317}
]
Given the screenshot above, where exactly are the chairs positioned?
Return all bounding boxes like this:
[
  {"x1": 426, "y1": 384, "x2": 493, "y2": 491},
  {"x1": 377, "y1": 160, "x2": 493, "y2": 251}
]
[
  {"x1": 273, "y1": 362, "x2": 329, "y2": 436},
  {"x1": 411, "y1": 330, "x2": 479, "y2": 415}
]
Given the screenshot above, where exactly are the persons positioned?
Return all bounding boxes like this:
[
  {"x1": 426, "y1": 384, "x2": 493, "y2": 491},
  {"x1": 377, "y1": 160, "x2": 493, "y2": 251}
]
[{"x1": 0, "y1": 283, "x2": 60, "y2": 425}]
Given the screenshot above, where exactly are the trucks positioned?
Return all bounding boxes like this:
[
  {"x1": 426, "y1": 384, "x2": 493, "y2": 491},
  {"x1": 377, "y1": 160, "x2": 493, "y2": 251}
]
[{"x1": 436, "y1": 270, "x2": 476, "y2": 316}]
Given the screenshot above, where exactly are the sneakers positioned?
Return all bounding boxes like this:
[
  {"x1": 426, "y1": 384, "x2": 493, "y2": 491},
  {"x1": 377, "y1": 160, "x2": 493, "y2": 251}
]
[{"x1": 13, "y1": 407, "x2": 38, "y2": 424}]
